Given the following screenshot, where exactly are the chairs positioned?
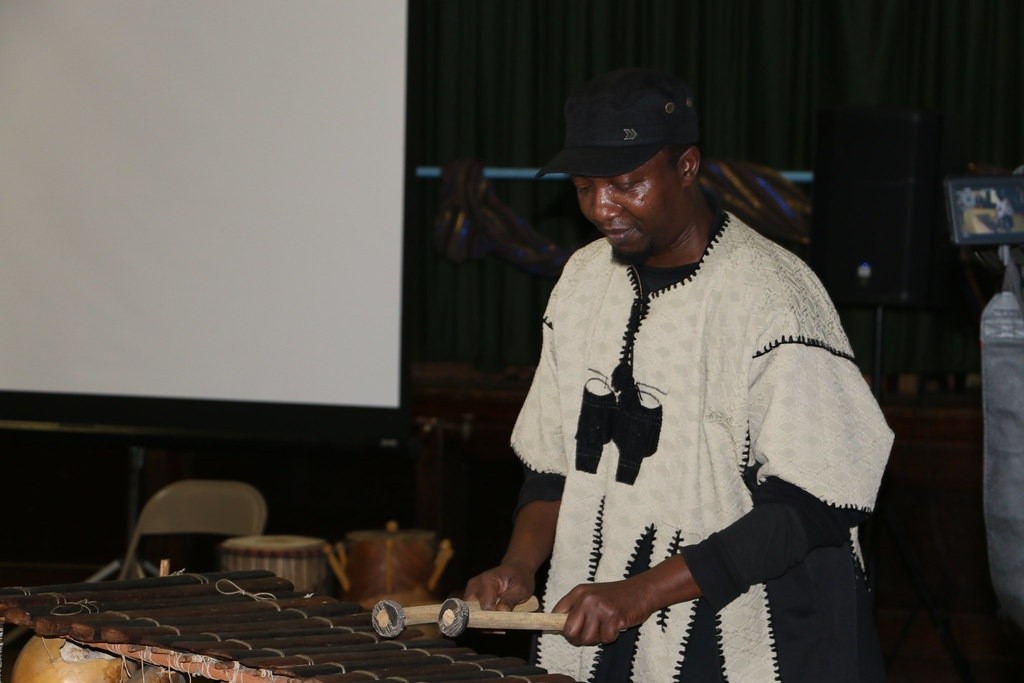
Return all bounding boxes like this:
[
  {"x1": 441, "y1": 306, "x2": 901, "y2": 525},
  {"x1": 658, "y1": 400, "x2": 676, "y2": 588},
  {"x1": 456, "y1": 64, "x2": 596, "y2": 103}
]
[{"x1": 118, "y1": 478, "x2": 268, "y2": 583}]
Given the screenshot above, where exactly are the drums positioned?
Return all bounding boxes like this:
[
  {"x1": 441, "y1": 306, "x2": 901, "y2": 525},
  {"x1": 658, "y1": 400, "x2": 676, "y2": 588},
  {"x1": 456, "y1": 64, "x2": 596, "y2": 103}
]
[
  {"x1": 219, "y1": 535, "x2": 328, "y2": 598},
  {"x1": 347, "y1": 528, "x2": 435, "y2": 609}
]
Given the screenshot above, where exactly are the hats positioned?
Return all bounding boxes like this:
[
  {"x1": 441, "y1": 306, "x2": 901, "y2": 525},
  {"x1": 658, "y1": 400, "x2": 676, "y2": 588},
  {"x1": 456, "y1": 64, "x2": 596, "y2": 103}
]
[{"x1": 533, "y1": 67, "x2": 699, "y2": 178}]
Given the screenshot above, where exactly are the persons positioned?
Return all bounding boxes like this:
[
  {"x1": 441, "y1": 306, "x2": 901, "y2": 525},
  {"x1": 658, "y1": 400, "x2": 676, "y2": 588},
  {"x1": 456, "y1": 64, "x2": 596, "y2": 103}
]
[{"x1": 464, "y1": 67, "x2": 896, "y2": 683}]
